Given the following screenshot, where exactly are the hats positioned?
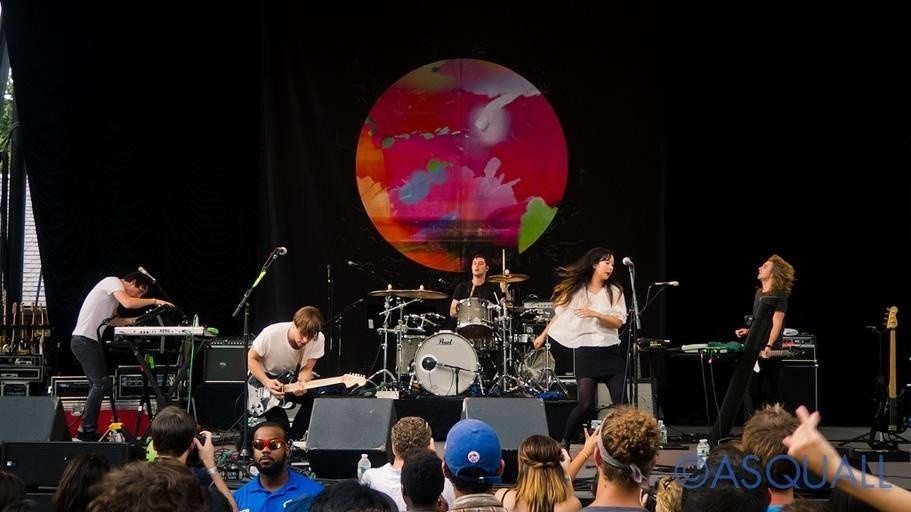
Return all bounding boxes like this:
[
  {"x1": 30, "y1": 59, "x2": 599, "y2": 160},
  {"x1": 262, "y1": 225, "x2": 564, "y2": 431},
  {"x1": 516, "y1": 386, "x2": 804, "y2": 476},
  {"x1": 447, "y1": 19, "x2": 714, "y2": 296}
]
[{"x1": 442, "y1": 418, "x2": 503, "y2": 484}]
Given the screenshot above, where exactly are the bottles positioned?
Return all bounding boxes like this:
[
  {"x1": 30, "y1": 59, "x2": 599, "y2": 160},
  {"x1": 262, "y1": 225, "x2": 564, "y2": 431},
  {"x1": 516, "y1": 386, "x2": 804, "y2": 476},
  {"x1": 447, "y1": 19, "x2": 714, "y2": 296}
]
[
  {"x1": 657, "y1": 419, "x2": 667, "y2": 444},
  {"x1": 697, "y1": 438, "x2": 711, "y2": 469},
  {"x1": 358, "y1": 453, "x2": 373, "y2": 481}
]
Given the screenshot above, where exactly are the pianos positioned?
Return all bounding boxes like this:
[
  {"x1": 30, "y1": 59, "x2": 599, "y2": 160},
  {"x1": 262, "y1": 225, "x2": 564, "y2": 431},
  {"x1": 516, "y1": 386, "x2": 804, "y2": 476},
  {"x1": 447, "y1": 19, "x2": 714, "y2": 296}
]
[{"x1": 114, "y1": 325, "x2": 218, "y2": 337}]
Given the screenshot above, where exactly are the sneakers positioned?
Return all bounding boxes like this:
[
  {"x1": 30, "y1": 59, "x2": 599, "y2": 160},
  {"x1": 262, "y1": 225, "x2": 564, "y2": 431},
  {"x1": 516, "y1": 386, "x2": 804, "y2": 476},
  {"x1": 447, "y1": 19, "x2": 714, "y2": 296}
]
[
  {"x1": 76, "y1": 427, "x2": 110, "y2": 443},
  {"x1": 559, "y1": 438, "x2": 572, "y2": 455}
]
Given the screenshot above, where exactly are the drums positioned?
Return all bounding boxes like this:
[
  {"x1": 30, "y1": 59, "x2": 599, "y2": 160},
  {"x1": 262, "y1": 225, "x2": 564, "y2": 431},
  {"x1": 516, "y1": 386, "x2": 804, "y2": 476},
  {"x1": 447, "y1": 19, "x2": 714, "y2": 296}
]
[
  {"x1": 414, "y1": 333, "x2": 479, "y2": 397},
  {"x1": 465, "y1": 338, "x2": 498, "y2": 352},
  {"x1": 455, "y1": 297, "x2": 491, "y2": 338},
  {"x1": 395, "y1": 335, "x2": 428, "y2": 376},
  {"x1": 508, "y1": 334, "x2": 537, "y2": 343}
]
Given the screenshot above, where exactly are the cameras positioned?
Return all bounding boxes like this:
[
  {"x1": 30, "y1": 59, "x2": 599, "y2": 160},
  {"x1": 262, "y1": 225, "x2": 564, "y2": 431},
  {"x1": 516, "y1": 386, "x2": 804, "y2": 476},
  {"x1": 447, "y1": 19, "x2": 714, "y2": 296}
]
[{"x1": 197, "y1": 436, "x2": 213, "y2": 446}]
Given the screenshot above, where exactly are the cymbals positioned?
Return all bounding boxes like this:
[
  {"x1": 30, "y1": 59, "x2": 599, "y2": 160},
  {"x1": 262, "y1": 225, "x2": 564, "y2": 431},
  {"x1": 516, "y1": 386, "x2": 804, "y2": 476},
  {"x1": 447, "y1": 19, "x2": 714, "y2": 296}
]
[
  {"x1": 486, "y1": 274, "x2": 527, "y2": 283},
  {"x1": 367, "y1": 290, "x2": 447, "y2": 300}
]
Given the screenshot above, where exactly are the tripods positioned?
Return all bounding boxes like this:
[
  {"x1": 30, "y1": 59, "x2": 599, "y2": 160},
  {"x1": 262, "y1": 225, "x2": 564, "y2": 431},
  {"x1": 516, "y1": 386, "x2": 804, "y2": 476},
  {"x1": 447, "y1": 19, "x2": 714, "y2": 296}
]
[
  {"x1": 529, "y1": 321, "x2": 568, "y2": 395},
  {"x1": 838, "y1": 335, "x2": 911, "y2": 447},
  {"x1": 349, "y1": 297, "x2": 398, "y2": 394},
  {"x1": 489, "y1": 319, "x2": 542, "y2": 394}
]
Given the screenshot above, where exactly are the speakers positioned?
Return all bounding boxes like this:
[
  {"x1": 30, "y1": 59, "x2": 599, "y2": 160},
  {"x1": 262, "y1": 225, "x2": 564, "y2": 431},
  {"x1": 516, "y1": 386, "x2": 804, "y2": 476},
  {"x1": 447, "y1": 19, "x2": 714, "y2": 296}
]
[
  {"x1": 0, "y1": 442, "x2": 137, "y2": 489},
  {"x1": 0, "y1": 396, "x2": 71, "y2": 443},
  {"x1": 771, "y1": 365, "x2": 818, "y2": 418},
  {"x1": 462, "y1": 397, "x2": 550, "y2": 485},
  {"x1": 197, "y1": 383, "x2": 248, "y2": 430},
  {"x1": 308, "y1": 398, "x2": 398, "y2": 478}
]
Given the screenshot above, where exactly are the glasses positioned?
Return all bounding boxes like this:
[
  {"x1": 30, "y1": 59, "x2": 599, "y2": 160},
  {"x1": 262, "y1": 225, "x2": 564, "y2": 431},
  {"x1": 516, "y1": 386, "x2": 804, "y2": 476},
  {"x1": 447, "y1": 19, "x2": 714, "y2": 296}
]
[{"x1": 252, "y1": 438, "x2": 287, "y2": 451}]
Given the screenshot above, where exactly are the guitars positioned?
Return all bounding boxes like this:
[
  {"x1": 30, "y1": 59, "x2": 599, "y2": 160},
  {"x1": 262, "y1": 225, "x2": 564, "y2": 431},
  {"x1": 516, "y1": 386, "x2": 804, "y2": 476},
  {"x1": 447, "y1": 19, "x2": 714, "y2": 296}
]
[
  {"x1": 0, "y1": 290, "x2": 44, "y2": 355},
  {"x1": 247, "y1": 369, "x2": 366, "y2": 417},
  {"x1": 873, "y1": 306, "x2": 911, "y2": 435}
]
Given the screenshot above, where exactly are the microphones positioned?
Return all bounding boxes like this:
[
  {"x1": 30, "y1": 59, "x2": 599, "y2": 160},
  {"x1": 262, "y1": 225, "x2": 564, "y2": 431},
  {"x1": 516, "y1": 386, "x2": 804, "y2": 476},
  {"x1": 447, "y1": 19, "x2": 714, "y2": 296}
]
[
  {"x1": 344, "y1": 259, "x2": 360, "y2": 267},
  {"x1": 138, "y1": 266, "x2": 156, "y2": 282},
  {"x1": 327, "y1": 264, "x2": 331, "y2": 282},
  {"x1": 279, "y1": 246, "x2": 288, "y2": 256},
  {"x1": 344, "y1": 299, "x2": 364, "y2": 309},
  {"x1": 655, "y1": 281, "x2": 679, "y2": 287},
  {"x1": 622, "y1": 256, "x2": 634, "y2": 266}
]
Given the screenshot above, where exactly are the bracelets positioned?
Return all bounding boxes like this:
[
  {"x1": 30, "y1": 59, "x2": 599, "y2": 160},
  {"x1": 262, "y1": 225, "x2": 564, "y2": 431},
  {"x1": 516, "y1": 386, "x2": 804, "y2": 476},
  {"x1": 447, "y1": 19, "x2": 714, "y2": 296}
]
[{"x1": 153, "y1": 296, "x2": 157, "y2": 306}]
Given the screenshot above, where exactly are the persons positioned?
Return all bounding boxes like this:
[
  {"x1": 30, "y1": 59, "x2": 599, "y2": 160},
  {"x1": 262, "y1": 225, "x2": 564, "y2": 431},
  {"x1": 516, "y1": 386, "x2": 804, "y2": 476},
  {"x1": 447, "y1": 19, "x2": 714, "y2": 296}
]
[
  {"x1": 441, "y1": 253, "x2": 506, "y2": 398},
  {"x1": 3, "y1": 405, "x2": 911, "y2": 510},
  {"x1": 69, "y1": 261, "x2": 175, "y2": 446},
  {"x1": 533, "y1": 246, "x2": 629, "y2": 455},
  {"x1": 235, "y1": 303, "x2": 327, "y2": 452},
  {"x1": 706, "y1": 252, "x2": 798, "y2": 453}
]
